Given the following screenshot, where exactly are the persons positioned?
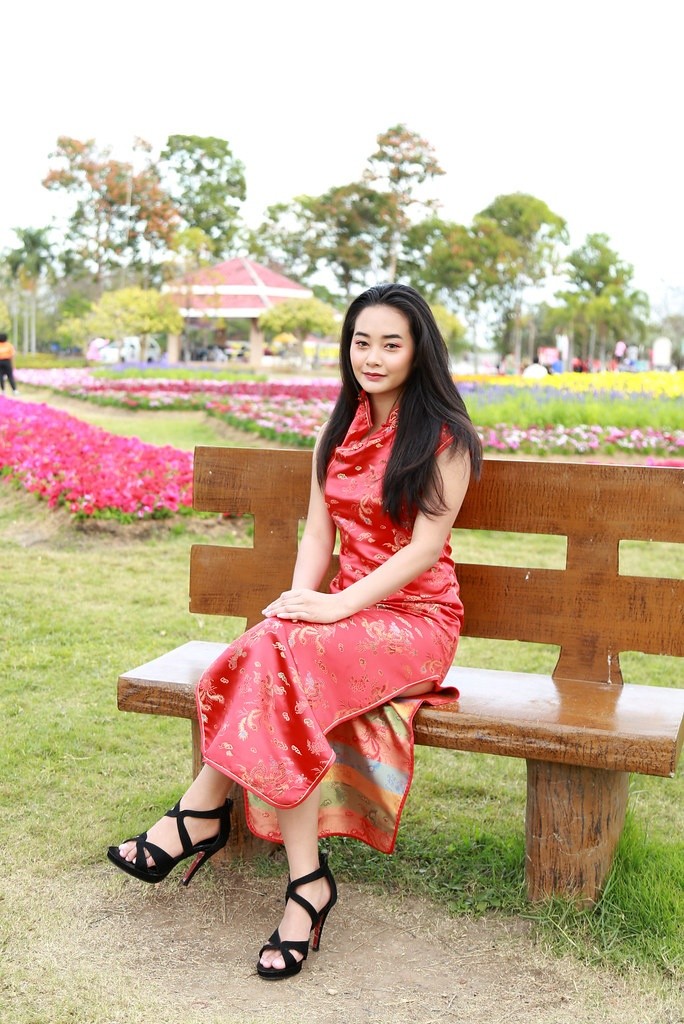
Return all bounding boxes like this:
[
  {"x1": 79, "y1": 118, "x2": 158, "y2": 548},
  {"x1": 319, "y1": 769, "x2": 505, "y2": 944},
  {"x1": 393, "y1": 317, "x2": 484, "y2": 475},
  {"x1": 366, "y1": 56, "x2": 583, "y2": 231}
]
[
  {"x1": 523, "y1": 357, "x2": 548, "y2": 377},
  {"x1": 107, "y1": 285, "x2": 482, "y2": 980},
  {"x1": 0, "y1": 333, "x2": 19, "y2": 394}
]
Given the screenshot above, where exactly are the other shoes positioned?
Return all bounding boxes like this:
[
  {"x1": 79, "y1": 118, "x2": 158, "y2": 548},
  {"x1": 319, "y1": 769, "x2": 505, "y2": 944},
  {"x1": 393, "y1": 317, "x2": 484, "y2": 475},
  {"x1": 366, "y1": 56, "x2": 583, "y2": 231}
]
[
  {"x1": 2, "y1": 390, "x2": 5, "y2": 392},
  {"x1": 13, "y1": 390, "x2": 19, "y2": 395}
]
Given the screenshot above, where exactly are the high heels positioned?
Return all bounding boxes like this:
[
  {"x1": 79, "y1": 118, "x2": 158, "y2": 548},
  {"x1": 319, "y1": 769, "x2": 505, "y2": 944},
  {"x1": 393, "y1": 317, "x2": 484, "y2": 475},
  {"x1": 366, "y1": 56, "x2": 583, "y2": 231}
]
[
  {"x1": 106, "y1": 798, "x2": 233, "y2": 886},
  {"x1": 257, "y1": 853, "x2": 337, "y2": 980}
]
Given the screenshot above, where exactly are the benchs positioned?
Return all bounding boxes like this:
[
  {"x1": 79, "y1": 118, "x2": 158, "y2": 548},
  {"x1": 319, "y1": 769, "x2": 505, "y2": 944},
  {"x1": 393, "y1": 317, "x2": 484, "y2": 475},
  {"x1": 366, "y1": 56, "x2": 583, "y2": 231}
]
[{"x1": 117, "y1": 444, "x2": 683, "y2": 906}]
